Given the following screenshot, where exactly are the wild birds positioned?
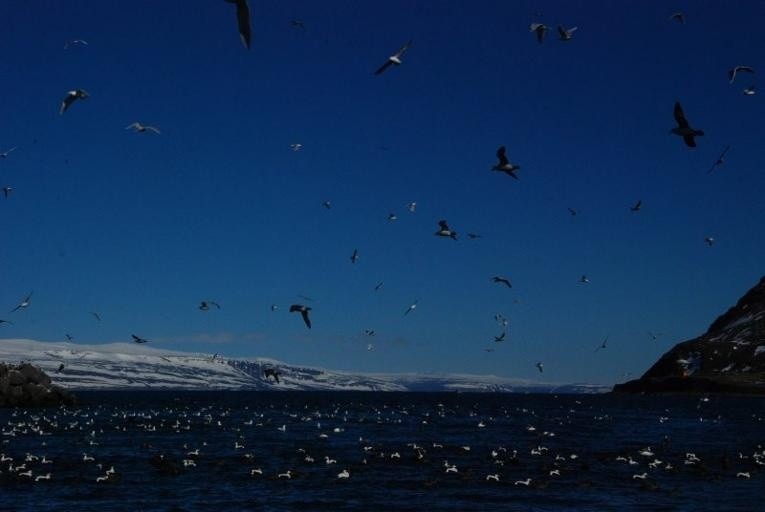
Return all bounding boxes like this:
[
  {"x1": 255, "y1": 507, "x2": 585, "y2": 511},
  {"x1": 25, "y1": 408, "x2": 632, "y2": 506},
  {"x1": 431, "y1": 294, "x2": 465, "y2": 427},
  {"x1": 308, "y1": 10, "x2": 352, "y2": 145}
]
[
  {"x1": 0, "y1": 146, "x2": 17, "y2": 199},
  {"x1": 58, "y1": 88, "x2": 90, "y2": 113},
  {"x1": 124, "y1": 122, "x2": 161, "y2": 136},
  {"x1": 374, "y1": 39, "x2": 414, "y2": 75},
  {"x1": 290, "y1": 19, "x2": 307, "y2": 33},
  {"x1": 728, "y1": 65, "x2": 755, "y2": 96},
  {"x1": 288, "y1": 140, "x2": 734, "y2": 266},
  {"x1": 528, "y1": 23, "x2": 578, "y2": 44},
  {"x1": 0, "y1": 274, "x2": 765, "y2": 512},
  {"x1": 224, "y1": 0, "x2": 252, "y2": 51},
  {"x1": 670, "y1": 100, "x2": 704, "y2": 148}
]
